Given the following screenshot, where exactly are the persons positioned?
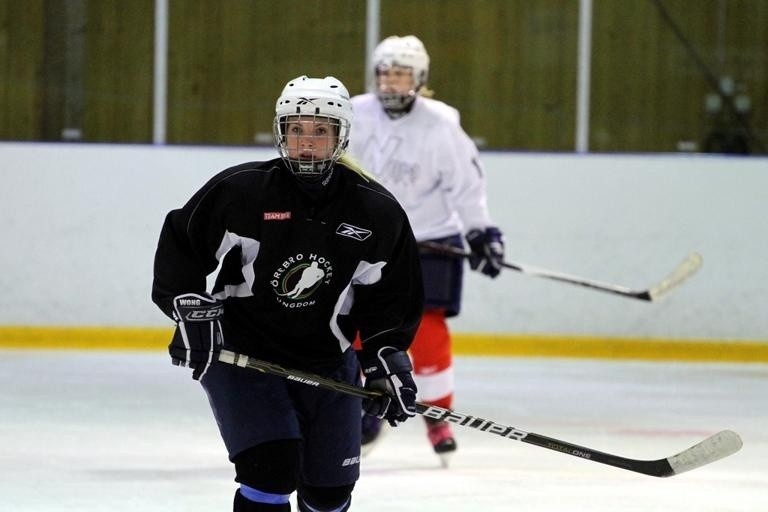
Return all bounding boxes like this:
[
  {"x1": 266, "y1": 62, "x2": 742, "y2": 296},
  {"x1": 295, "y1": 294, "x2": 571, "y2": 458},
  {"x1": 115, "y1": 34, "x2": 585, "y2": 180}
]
[
  {"x1": 343, "y1": 33, "x2": 504, "y2": 450},
  {"x1": 149, "y1": 72, "x2": 423, "y2": 511}
]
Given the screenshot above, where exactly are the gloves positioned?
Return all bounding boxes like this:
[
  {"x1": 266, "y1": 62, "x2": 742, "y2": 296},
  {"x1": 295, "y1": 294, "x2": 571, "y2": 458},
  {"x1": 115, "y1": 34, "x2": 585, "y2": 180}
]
[
  {"x1": 356, "y1": 345, "x2": 416, "y2": 427},
  {"x1": 169, "y1": 293, "x2": 224, "y2": 382},
  {"x1": 464, "y1": 226, "x2": 504, "y2": 278}
]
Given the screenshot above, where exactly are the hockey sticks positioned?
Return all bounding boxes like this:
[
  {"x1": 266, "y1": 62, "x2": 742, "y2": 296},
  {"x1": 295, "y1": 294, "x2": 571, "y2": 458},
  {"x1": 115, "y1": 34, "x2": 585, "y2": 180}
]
[
  {"x1": 419, "y1": 241, "x2": 702, "y2": 302},
  {"x1": 218, "y1": 349, "x2": 742, "y2": 476}
]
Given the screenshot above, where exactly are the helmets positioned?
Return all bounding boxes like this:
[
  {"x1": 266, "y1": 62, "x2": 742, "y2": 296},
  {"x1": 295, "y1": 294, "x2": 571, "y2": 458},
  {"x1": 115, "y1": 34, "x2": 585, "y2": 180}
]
[
  {"x1": 272, "y1": 75, "x2": 352, "y2": 176},
  {"x1": 368, "y1": 34, "x2": 431, "y2": 111}
]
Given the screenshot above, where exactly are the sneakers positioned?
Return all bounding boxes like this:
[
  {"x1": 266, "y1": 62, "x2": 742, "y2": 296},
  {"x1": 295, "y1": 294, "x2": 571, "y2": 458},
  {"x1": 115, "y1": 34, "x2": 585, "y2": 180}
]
[{"x1": 423, "y1": 416, "x2": 455, "y2": 453}]
